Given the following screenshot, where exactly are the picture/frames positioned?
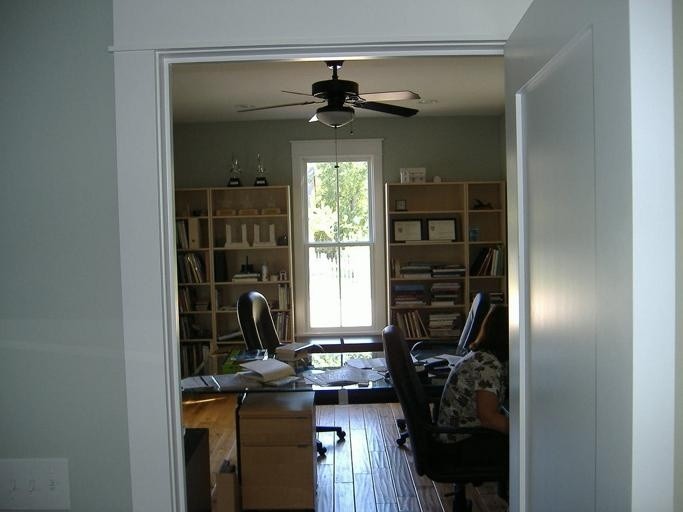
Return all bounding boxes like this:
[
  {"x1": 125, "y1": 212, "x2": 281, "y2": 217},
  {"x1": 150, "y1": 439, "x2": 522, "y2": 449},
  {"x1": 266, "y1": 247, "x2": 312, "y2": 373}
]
[
  {"x1": 390, "y1": 218, "x2": 423, "y2": 243},
  {"x1": 425, "y1": 218, "x2": 458, "y2": 242}
]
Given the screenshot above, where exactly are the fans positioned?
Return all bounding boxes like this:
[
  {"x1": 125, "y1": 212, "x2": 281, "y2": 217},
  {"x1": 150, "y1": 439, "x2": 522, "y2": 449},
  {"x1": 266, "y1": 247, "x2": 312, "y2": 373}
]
[{"x1": 235, "y1": 61, "x2": 422, "y2": 130}]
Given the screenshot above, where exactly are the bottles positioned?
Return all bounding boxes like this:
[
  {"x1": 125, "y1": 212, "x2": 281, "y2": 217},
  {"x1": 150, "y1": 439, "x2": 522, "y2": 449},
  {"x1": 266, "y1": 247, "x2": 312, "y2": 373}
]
[{"x1": 261, "y1": 260, "x2": 269, "y2": 281}]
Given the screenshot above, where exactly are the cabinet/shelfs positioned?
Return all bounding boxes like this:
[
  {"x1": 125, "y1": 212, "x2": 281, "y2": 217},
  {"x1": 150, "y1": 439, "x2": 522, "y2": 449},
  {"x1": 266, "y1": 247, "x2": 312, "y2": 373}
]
[
  {"x1": 210, "y1": 185, "x2": 291, "y2": 382},
  {"x1": 173, "y1": 187, "x2": 211, "y2": 378},
  {"x1": 384, "y1": 181, "x2": 465, "y2": 344},
  {"x1": 182, "y1": 427, "x2": 211, "y2": 511},
  {"x1": 465, "y1": 181, "x2": 508, "y2": 360},
  {"x1": 235, "y1": 386, "x2": 317, "y2": 511}
]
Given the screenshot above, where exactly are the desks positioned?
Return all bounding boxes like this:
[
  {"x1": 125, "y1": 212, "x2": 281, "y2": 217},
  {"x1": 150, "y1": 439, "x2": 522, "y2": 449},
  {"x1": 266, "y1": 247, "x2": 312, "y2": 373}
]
[{"x1": 242, "y1": 349, "x2": 466, "y2": 392}]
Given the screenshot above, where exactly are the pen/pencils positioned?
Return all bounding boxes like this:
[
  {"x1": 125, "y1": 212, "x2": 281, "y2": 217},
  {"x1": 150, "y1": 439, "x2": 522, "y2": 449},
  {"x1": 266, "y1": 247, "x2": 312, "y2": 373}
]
[{"x1": 199, "y1": 375, "x2": 210, "y2": 386}]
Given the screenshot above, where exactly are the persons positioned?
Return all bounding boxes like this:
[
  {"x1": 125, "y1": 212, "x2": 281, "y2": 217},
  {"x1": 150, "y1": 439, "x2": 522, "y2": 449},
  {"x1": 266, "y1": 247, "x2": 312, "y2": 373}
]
[{"x1": 436, "y1": 304, "x2": 509, "y2": 510}]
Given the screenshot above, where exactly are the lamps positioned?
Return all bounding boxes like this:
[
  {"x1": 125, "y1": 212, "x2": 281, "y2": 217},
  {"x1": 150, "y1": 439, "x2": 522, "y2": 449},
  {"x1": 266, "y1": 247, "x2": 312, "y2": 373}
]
[{"x1": 313, "y1": 104, "x2": 355, "y2": 129}]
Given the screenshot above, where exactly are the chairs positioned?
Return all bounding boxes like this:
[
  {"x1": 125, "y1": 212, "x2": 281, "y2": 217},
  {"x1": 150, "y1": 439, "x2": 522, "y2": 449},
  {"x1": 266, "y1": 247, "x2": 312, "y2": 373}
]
[
  {"x1": 236, "y1": 290, "x2": 346, "y2": 456},
  {"x1": 392, "y1": 291, "x2": 484, "y2": 447},
  {"x1": 381, "y1": 325, "x2": 509, "y2": 511}
]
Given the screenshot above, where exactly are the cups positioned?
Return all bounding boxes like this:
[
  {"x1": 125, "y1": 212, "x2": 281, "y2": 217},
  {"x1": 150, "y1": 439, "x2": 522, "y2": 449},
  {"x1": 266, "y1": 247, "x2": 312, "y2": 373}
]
[
  {"x1": 270, "y1": 275, "x2": 278, "y2": 281},
  {"x1": 471, "y1": 224, "x2": 480, "y2": 241}
]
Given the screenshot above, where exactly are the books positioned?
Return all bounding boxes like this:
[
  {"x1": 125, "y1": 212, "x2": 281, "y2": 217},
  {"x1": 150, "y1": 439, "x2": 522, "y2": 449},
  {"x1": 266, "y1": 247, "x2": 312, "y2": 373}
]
[
  {"x1": 176, "y1": 217, "x2": 210, "y2": 380},
  {"x1": 275, "y1": 342, "x2": 314, "y2": 360},
  {"x1": 236, "y1": 358, "x2": 291, "y2": 383},
  {"x1": 231, "y1": 278, "x2": 259, "y2": 283},
  {"x1": 390, "y1": 245, "x2": 503, "y2": 339},
  {"x1": 270, "y1": 284, "x2": 293, "y2": 342}
]
